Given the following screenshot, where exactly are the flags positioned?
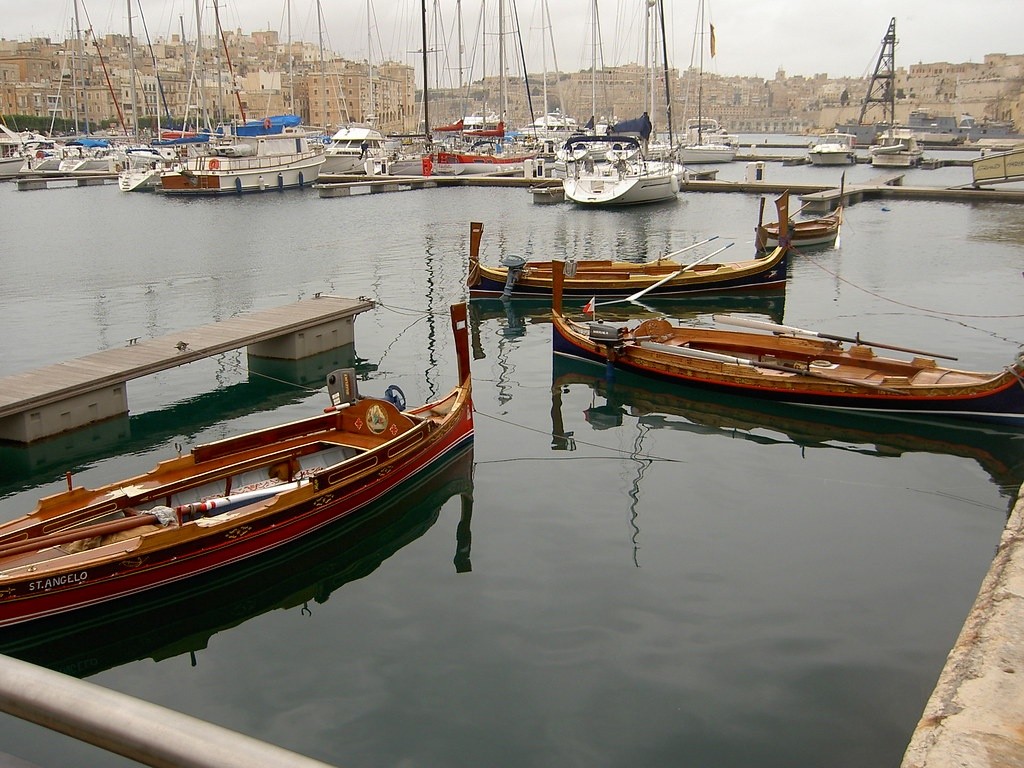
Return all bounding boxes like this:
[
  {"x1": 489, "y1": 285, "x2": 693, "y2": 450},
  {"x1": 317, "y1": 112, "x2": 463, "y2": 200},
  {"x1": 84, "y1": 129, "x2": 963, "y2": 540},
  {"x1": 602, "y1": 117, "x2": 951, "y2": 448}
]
[{"x1": 582, "y1": 297, "x2": 594, "y2": 316}]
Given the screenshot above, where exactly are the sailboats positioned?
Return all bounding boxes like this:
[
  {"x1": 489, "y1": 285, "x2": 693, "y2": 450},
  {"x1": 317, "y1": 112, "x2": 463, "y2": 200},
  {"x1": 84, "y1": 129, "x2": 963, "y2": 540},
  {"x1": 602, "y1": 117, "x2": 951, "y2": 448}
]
[{"x1": 1, "y1": 2, "x2": 739, "y2": 212}]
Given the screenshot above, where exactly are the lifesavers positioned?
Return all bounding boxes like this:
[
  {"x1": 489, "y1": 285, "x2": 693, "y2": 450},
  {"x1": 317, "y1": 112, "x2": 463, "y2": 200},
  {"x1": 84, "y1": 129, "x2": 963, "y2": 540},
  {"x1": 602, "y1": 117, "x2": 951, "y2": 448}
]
[
  {"x1": 262, "y1": 119, "x2": 271, "y2": 129},
  {"x1": 209, "y1": 159, "x2": 219, "y2": 171}
]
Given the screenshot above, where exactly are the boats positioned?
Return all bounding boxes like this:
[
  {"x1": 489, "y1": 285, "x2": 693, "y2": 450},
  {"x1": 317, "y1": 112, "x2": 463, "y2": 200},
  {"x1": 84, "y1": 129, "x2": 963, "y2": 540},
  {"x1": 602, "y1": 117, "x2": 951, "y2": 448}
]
[
  {"x1": 754, "y1": 170, "x2": 846, "y2": 257},
  {"x1": 867, "y1": 127, "x2": 926, "y2": 168},
  {"x1": 0, "y1": 303, "x2": 478, "y2": 650},
  {"x1": 466, "y1": 189, "x2": 790, "y2": 302},
  {"x1": 544, "y1": 259, "x2": 1024, "y2": 423},
  {"x1": 806, "y1": 131, "x2": 856, "y2": 168}
]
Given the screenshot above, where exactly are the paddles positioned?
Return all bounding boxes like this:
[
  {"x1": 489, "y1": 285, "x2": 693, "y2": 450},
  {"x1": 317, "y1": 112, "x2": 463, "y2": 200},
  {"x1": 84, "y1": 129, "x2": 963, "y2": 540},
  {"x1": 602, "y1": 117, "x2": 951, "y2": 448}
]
[
  {"x1": 580, "y1": 235, "x2": 737, "y2": 310},
  {"x1": 710, "y1": 314, "x2": 960, "y2": 361},
  {"x1": 0, "y1": 477, "x2": 310, "y2": 559},
  {"x1": 786, "y1": 201, "x2": 812, "y2": 219},
  {"x1": 833, "y1": 205, "x2": 843, "y2": 251},
  {"x1": 641, "y1": 340, "x2": 912, "y2": 395}
]
